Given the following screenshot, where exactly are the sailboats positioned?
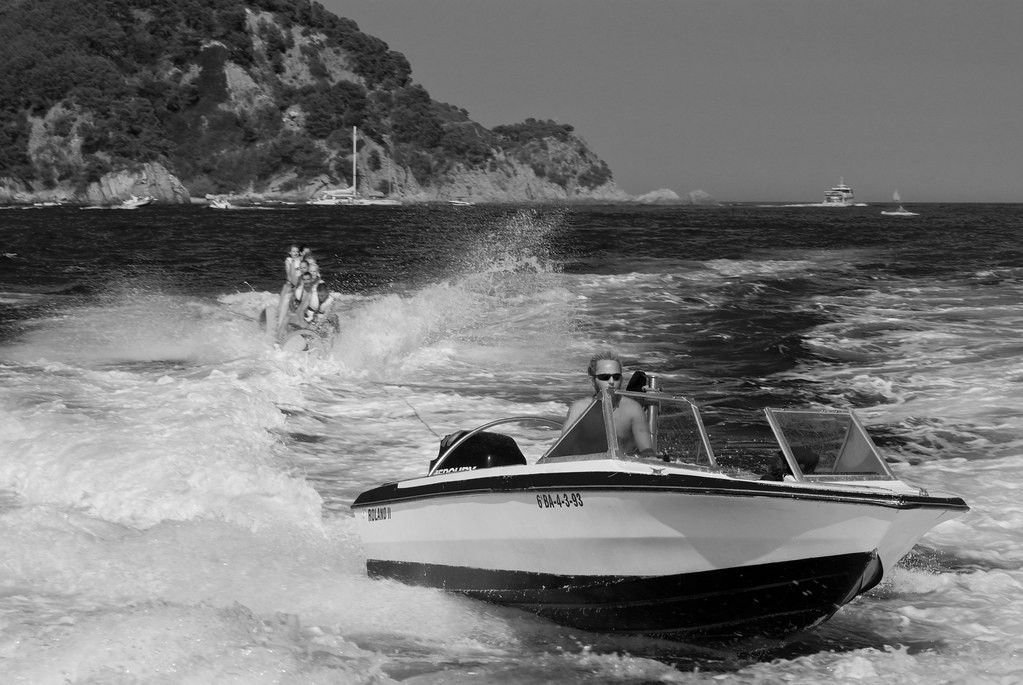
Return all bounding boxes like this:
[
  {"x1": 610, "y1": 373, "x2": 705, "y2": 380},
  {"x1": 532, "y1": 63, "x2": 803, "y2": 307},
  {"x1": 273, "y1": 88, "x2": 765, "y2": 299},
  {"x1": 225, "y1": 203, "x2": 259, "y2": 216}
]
[{"x1": 305, "y1": 126, "x2": 404, "y2": 207}]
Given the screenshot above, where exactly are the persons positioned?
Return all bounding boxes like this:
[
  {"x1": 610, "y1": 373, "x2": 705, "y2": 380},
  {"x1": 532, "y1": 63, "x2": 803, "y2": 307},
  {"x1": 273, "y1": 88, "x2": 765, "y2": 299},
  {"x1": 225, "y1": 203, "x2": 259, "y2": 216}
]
[
  {"x1": 284, "y1": 245, "x2": 335, "y2": 336},
  {"x1": 562, "y1": 352, "x2": 657, "y2": 462}
]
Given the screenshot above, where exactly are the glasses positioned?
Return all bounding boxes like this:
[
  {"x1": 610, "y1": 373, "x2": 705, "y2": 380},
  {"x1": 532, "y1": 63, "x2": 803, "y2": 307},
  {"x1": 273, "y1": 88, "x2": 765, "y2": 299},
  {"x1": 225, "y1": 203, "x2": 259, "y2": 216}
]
[{"x1": 594, "y1": 374, "x2": 623, "y2": 381}]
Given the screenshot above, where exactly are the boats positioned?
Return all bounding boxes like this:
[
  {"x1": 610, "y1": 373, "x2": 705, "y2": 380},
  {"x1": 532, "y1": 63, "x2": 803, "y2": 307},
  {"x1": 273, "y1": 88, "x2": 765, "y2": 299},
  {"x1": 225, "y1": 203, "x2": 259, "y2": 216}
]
[
  {"x1": 259, "y1": 307, "x2": 340, "y2": 362},
  {"x1": 209, "y1": 200, "x2": 231, "y2": 210},
  {"x1": 821, "y1": 176, "x2": 854, "y2": 208},
  {"x1": 881, "y1": 205, "x2": 920, "y2": 217},
  {"x1": 449, "y1": 200, "x2": 476, "y2": 207},
  {"x1": 350, "y1": 371, "x2": 972, "y2": 671},
  {"x1": 123, "y1": 193, "x2": 158, "y2": 207}
]
